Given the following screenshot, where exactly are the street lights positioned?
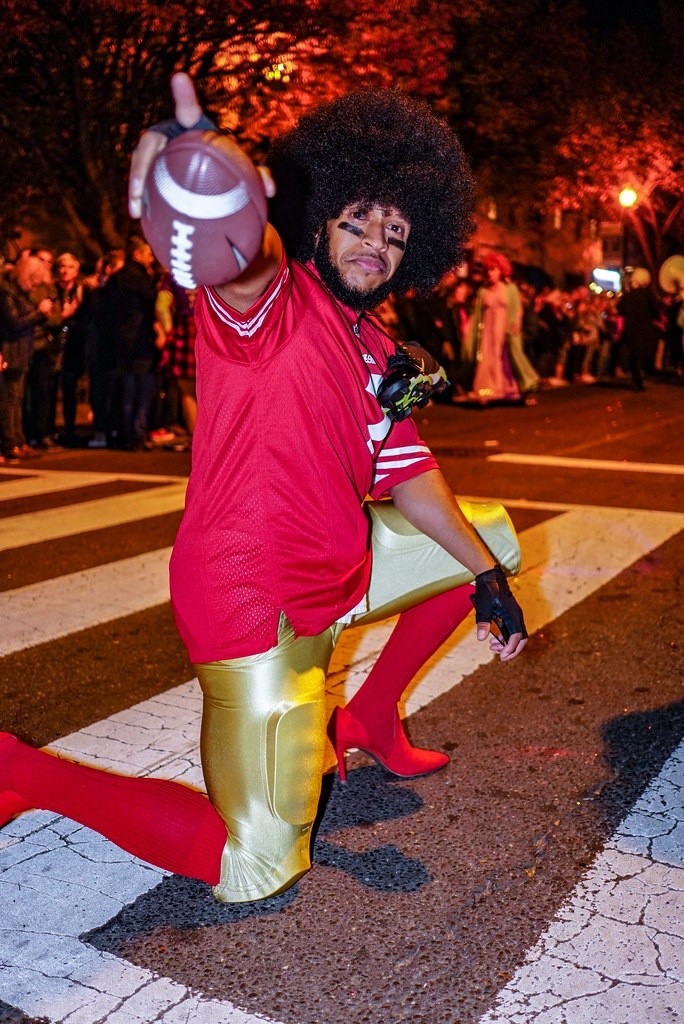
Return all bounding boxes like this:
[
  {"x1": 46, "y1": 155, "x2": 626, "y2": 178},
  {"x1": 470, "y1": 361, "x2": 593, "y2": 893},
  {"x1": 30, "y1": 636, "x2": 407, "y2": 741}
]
[{"x1": 619, "y1": 185, "x2": 639, "y2": 380}]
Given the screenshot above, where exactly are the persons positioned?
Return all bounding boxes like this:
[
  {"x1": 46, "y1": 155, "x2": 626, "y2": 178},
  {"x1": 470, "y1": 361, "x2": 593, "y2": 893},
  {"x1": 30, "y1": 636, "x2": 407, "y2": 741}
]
[
  {"x1": 0, "y1": 223, "x2": 684, "y2": 465},
  {"x1": 0, "y1": 73, "x2": 529, "y2": 905}
]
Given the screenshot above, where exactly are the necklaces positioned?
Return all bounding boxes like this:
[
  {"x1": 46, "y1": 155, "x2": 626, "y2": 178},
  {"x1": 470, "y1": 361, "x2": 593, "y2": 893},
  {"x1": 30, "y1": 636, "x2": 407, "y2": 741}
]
[{"x1": 352, "y1": 311, "x2": 365, "y2": 338}]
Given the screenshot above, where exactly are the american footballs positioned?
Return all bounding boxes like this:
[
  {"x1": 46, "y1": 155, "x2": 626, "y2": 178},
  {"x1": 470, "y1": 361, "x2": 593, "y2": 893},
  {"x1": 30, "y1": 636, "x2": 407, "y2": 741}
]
[{"x1": 129, "y1": 122, "x2": 274, "y2": 291}]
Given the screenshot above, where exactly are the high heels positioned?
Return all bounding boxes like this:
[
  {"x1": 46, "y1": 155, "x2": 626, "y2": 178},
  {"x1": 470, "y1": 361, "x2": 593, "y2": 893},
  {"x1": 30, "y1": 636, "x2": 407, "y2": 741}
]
[
  {"x1": 327, "y1": 704, "x2": 449, "y2": 785},
  {"x1": 0, "y1": 732, "x2": 30, "y2": 832}
]
